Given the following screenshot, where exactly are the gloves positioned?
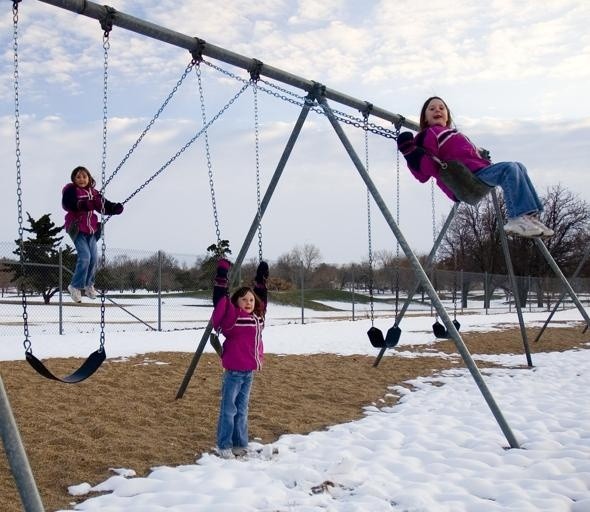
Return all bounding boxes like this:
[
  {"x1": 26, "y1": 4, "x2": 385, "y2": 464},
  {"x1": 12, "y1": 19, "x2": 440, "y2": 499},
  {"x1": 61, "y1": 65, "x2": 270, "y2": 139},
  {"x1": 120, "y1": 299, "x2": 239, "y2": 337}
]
[
  {"x1": 80, "y1": 200, "x2": 94, "y2": 211},
  {"x1": 112, "y1": 203, "x2": 123, "y2": 214},
  {"x1": 397, "y1": 132, "x2": 417, "y2": 166},
  {"x1": 217, "y1": 259, "x2": 230, "y2": 277},
  {"x1": 255, "y1": 262, "x2": 268, "y2": 283}
]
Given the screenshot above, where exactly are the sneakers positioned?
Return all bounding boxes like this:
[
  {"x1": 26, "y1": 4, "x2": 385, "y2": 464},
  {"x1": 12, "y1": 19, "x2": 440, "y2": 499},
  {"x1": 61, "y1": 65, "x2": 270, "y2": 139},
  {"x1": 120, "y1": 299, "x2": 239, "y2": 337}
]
[
  {"x1": 216, "y1": 445, "x2": 249, "y2": 459},
  {"x1": 503, "y1": 214, "x2": 554, "y2": 238},
  {"x1": 84, "y1": 286, "x2": 96, "y2": 299},
  {"x1": 68, "y1": 284, "x2": 81, "y2": 303}
]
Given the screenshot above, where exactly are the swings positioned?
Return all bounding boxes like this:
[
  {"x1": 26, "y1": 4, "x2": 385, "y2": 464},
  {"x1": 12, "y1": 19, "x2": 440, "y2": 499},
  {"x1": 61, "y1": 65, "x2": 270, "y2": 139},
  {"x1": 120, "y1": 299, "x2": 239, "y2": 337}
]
[
  {"x1": 362, "y1": 110, "x2": 402, "y2": 348},
  {"x1": 431, "y1": 175, "x2": 460, "y2": 338},
  {"x1": 68, "y1": 36, "x2": 263, "y2": 243},
  {"x1": 11, "y1": 0, "x2": 116, "y2": 383},
  {"x1": 188, "y1": 36, "x2": 263, "y2": 358},
  {"x1": 197, "y1": 53, "x2": 495, "y2": 206}
]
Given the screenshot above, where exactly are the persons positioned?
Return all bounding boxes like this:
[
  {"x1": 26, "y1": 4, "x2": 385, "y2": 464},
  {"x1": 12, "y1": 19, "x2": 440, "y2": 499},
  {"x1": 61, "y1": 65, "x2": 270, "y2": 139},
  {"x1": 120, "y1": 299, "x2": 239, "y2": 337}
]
[
  {"x1": 397, "y1": 94, "x2": 559, "y2": 242},
  {"x1": 209, "y1": 257, "x2": 270, "y2": 447},
  {"x1": 60, "y1": 166, "x2": 124, "y2": 304}
]
